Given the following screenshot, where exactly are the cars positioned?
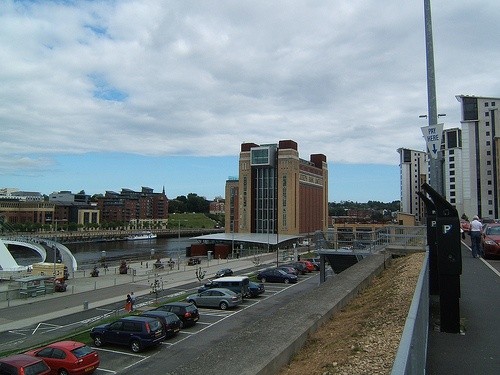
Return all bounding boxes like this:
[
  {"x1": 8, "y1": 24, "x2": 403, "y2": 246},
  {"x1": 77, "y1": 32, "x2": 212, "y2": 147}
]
[
  {"x1": 0, "y1": 353, "x2": 52, "y2": 375},
  {"x1": 271, "y1": 258, "x2": 320, "y2": 276},
  {"x1": 256, "y1": 269, "x2": 298, "y2": 284},
  {"x1": 215, "y1": 268, "x2": 233, "y2": 279},
  {"x1": 477, "y1": 223, "x2": 500, "y2": 260},
  {"x1": 184, "y1": 288, "x2": 243, "y2": 311},
  {"x1": 460, "y1": 219, "x2": 471, "y2": 232},
  {"x1": 22, "y1": 340, "x2": 100, "y2": 375},
  {"x1": 249, "y1": 282, "x2": 265, "y2": 298}
]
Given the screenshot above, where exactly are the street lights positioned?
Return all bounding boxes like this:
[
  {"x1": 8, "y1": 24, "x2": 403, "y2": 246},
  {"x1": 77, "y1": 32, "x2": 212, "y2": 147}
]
[{"x1": 53, "y1": 219, "x2": 68, "y2": 276}]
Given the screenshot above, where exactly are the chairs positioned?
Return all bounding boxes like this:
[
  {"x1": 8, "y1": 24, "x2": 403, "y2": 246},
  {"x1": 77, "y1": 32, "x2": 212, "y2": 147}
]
[{"x1": 173, "y1": 307, "x2": 179, "y2": 311}]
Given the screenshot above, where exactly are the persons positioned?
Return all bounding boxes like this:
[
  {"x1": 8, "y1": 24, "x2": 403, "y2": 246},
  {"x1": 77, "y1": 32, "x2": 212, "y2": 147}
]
[
  {"x1": 468, "y1": 215, "x2": 482, "y2": 258},
  {"x1": 126, "y1": 291, "x2": 135, "y2": 313}
]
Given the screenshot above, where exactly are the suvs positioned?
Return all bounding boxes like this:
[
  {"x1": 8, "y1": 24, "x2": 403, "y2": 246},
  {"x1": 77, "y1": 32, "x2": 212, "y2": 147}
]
[
  {"x1": 197, "y1": 276, "x2": 250, "y2": 299},
  {"x1": 146, "y1": 302, "x2": 200, "y2": 329},
  {"x1": 89, "y1": 315, "x2": 167, "y2": 354},
  {"x1": 132, "y1": 311, "x2": 183, "y2": 340}
]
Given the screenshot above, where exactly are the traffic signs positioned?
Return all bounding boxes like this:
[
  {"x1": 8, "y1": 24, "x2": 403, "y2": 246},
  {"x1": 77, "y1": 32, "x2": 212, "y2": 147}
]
[{"x1": 420, "y1": 123, "x2": 443, "y2": 160}]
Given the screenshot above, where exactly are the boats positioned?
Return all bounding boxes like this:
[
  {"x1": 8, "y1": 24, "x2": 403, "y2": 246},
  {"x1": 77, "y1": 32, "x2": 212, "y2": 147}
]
[{"x1": 133, "y1": 231, "x2": 157, "y2": 240}]
